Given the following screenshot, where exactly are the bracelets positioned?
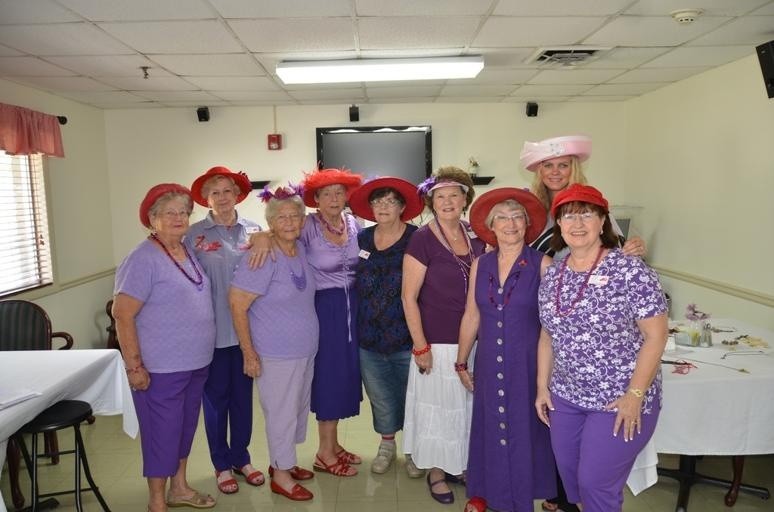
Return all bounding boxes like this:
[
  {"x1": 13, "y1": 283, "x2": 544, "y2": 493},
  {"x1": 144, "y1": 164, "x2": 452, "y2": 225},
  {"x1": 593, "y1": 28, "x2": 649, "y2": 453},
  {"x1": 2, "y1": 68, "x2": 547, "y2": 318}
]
[
  {"x1": 456, "y1": 364, "x2": 467, "y2": 372},
  {"x1": 412, "y1": 342, "x2": 431, "y2": 355},
  {"x1": 629, "y1": 387, "x2": 644, "y2": 399},
  {"x1": 125, "y1": 364, "x2": 143, "y2": 374}
]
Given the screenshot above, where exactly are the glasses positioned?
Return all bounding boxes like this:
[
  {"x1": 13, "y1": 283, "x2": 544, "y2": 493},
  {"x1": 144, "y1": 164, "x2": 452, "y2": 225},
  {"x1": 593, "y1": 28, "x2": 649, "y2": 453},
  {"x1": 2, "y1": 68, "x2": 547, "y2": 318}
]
[
  {"x1": 564, "y1": 213, "x2": 592, "y2": 223},
  {"x1": 370, "y1": 198, "x2": 399, "y2": 206},
  {"x1": 494, "y1": 215, "x2": 524, "y2": 222}
]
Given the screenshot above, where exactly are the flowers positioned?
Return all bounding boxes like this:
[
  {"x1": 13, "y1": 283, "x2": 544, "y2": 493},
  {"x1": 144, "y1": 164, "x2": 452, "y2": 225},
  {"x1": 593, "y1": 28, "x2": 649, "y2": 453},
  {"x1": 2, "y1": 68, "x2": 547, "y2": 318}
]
[
  {"x1": 467, "y1": 156, "x2": 481, "y2": 168},
  {"x1": 685, "y1": 303, "x2": 710, "y2": 320}
]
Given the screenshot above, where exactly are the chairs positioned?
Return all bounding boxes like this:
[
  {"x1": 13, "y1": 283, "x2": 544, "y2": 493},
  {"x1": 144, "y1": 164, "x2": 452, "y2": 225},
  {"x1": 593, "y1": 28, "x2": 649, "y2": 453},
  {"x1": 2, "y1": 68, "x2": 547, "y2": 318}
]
[
  {"x1": 0, "y1": 301, "x2": 72, "y2": 508},
  {"x1": 105, "y1": 300, "x2": 120, "y2": 348}
]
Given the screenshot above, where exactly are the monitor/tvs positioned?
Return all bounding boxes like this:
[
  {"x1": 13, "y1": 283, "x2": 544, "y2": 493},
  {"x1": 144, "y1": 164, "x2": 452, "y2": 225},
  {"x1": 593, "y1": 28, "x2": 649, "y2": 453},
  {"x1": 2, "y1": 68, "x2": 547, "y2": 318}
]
[{"x1": 315, "y1": 125, "x2": 433, "y2": 188}]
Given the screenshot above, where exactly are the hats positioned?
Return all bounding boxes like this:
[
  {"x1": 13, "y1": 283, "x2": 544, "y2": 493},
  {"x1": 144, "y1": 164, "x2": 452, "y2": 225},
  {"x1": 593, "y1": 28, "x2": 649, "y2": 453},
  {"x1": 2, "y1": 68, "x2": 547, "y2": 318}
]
[
  {"x1": 302, "y1": 169, "x2": 360, "y2": 208},
  {"x1": 140, "y1": 184, "x2": 193, "y2": 227},
  {"x1": 551, "y1": 184, "x2": 608, "y2": 216},
  {"x1": 417, "y1": 174, "x2": 469, "y2": 197},
  {"x1": 521, "y1": 135, "x2": 592, "y2": 171},
  {"x1": 469, "y1": 188, "x2": 547, "y2": 246},
  {"x1": 191, "y1": 166, "x2": 252, "y2": 207},
  {"x1": 349, "y1": 177, "x2": 424, "y2": 221}
]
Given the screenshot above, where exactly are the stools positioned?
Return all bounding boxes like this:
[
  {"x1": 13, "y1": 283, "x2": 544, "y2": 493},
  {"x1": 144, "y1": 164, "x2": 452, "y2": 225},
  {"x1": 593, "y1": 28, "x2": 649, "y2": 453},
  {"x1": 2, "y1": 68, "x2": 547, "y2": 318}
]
[{"x1": 11, "y1": 400, "x2": 110, "y2": 512}]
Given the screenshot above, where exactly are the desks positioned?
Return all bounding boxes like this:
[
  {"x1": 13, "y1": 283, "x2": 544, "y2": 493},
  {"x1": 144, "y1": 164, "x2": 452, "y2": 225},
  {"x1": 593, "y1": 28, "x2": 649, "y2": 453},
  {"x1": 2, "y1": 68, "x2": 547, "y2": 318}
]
[
  {"x1": 625, "y1": 313, "x2": 774, "y2": 511},
  {"x1": 0, "y1": 348, "x2": 122, "y2": 512}
]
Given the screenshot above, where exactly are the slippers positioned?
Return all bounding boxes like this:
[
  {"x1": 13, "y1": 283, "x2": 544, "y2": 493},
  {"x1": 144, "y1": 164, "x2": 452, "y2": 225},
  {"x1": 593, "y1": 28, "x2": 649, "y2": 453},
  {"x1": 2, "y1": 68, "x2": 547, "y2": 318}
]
[{"x1": 168, "y1": 491, "x2": 217, "y2": 509}]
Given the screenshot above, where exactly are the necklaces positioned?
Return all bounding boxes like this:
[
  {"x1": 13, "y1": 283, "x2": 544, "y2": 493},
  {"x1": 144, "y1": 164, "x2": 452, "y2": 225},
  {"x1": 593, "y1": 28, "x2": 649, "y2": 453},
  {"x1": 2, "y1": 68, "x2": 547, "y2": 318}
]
[
  {"x1": 431, "y1": 217, "x2": 475, "y2": 295},
  {"x1": 488, "y1": 272, "x2": 521, "y2": 311},
  {"x1": 148, "y1": 233, "x2": 204, "y2": 292},
  {"x1": 556, "y1": 244, "x2": 604, "y2": 316},
  {"x1": 317, "y1": 209, "x2": 345, "y2": 236},
  {"x1": 273, "y1": 237, "x2": 307, "y2": 292}
]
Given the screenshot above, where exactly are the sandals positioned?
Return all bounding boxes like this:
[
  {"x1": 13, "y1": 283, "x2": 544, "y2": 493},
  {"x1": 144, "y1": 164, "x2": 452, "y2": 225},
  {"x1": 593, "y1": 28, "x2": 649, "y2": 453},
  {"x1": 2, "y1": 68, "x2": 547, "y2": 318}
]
[
  {"x1": 235, "y1": 464, "x2": 265, "y2": 486},
  {"x1": 215, "y1": 468, "x2": 239, "y2": 495},
  {"x1": 335, "y1": 446, "x2": 361, "y2": 464},
  {"x1": 313, "y1": 453, "x2": 358, "y2": 476}
]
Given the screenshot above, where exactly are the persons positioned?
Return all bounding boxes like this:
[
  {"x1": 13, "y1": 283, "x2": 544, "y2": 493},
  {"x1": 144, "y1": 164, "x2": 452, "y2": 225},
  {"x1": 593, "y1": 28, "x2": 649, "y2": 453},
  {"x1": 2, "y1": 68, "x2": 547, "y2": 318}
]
[
  {"x1": 401, "y1": 166, "x2": 496, "y2": 505},
  {"x1": 536, "y1": 183, "x2": 669, "y2": 512},
  {"x1": 229, "y1": 195, "x2": 321, "y2": 500},
  {"x1": 455, "y1": 199, "x2": 554, "y2": 512},
  {"x1": 247, "y1": 183, "x2": 362, "y2": 476},
  {"x1": 358, "y1": 188, "x2": 418, "y2": 475},
  {"x1": 185, "y1": 174, "x2": 262, "y2": 493},
  {"x1": 529, "y1": 155, "x2": 646, "y2": 258},
  {"x1": 112, "y1": 183, "x2": 215, "y2": 512}
]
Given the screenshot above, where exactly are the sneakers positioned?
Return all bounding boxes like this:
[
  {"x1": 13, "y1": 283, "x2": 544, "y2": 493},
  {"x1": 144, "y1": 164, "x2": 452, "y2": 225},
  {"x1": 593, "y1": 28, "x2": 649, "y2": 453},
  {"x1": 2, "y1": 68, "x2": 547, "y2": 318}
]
[
  {"x1": 405, "y1": 453, "x2": 426, "y2": 478},
  {"x1": 371, "y1": 442, "x2": 396, "y2": 473}
]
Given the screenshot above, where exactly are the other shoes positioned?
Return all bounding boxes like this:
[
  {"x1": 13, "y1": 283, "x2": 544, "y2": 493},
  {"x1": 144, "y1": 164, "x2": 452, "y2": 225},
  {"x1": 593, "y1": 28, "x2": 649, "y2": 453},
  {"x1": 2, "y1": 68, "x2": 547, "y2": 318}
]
[
  {"x1": 271, "y1": 479, "x2": 313, "y2": 501},
  {"x1": 268, "y1": 464, "x2": 314, "y2": 480},
  {"x1": 464, "y1": 496, "x2": 488, "y2": 510},
  {"x1": 446, "y1": 473, "x2": 466, "y2": 486},
  {"x1": 427, "y1": 472, "x2": 454, "y2": 504},
  {"x1": 541, "y1": 497, "x2": 559, "y2": 510}
]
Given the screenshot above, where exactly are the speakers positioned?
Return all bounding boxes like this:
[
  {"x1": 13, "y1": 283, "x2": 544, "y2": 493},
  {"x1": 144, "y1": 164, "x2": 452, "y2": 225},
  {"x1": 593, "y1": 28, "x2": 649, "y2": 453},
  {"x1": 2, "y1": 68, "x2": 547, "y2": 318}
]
[
  {"x1": 197, "y1": 107, "x2": 210, "y2": 122},
  {"x1": 526, "y1": 102, "x2": 538, "y2": 117},
  {"x1": 350, "y1": 106, "x2": 360, "y2": 121},
  {"x1": 754, "y1": 40, "x2": 774, "y2": 98}
]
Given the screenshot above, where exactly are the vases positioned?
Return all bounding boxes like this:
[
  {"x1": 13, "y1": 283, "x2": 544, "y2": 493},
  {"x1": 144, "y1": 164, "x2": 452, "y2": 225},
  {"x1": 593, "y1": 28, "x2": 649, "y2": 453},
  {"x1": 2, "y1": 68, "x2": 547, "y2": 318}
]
[
  {"x1": 469, "y1": 168, "x2": 477, "y2": 173},
  {"x1": 690, "y1": 322, "x2": 704, "y2": 333}
]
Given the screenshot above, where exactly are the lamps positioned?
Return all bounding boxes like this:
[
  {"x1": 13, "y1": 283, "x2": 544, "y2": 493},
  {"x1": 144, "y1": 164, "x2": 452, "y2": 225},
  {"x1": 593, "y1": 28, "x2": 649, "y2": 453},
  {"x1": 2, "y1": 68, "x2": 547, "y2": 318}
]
[{"x1": 274, "y1": 53, "x2": 484, "y2": 85}]
